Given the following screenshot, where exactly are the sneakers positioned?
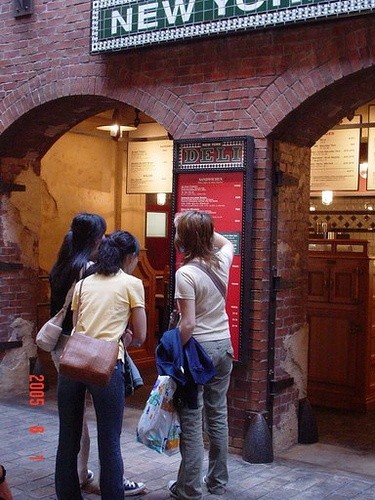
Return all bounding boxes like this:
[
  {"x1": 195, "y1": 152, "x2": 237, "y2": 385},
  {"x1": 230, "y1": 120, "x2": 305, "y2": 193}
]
[{"x1": 123, "y1": 476, "x2": 146, "y2": 496}]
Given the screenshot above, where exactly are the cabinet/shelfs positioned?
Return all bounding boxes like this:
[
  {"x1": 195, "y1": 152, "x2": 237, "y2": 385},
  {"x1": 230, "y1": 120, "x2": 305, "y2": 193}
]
[{"x1": 306, "y1": 238, "x2": 375, "y2": 414}]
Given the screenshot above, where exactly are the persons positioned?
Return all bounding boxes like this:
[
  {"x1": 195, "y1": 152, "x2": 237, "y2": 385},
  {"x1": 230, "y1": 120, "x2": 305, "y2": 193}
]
[
  {"x1": 54, "y1": 230, "x2": 145, "y2": 500},
  {"x1": 46, "y1": 212, "x2": 109, "y2": 491},
  {"x1": 159, "y1": 209, "x2": 237, "y2": 500}
]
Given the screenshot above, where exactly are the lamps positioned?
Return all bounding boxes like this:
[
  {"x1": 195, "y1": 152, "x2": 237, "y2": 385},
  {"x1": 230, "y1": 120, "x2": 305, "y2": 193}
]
[{"x1": 96, "y1": 106, "x2": 138, "y2": 141}]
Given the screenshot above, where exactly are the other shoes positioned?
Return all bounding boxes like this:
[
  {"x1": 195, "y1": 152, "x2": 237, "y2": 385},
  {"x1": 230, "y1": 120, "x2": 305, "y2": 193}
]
[
  {"x1": 167, "y1": 478, "x2": 204, "y2": 500},
  {"x1": 78, "y1": 473, "x2": 104, "y2": 495},
  {"x1": 203, "y1": 475, "x2": 208, "y2": 484}
]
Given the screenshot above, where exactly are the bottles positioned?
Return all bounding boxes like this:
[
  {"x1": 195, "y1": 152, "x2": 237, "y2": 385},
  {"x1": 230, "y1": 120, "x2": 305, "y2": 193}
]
[{"x1": 168, "y1": 309, "x2": 178, "y2": 331}]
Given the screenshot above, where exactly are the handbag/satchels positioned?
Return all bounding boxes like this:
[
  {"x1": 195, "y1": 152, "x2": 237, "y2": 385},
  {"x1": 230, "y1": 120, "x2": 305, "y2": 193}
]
[
  {"x1": 58, "y1": 331, "x2": 120, "y2": 385},
  {"x1": 36, "y1": 308, "x2": 67, "y2": 352},
  {"x1": 161, "y1": 412, "x2": 180, "y2": 457},
  {"x1": 138, "y1": 374, "x2": 177, "y2": 455}
]
